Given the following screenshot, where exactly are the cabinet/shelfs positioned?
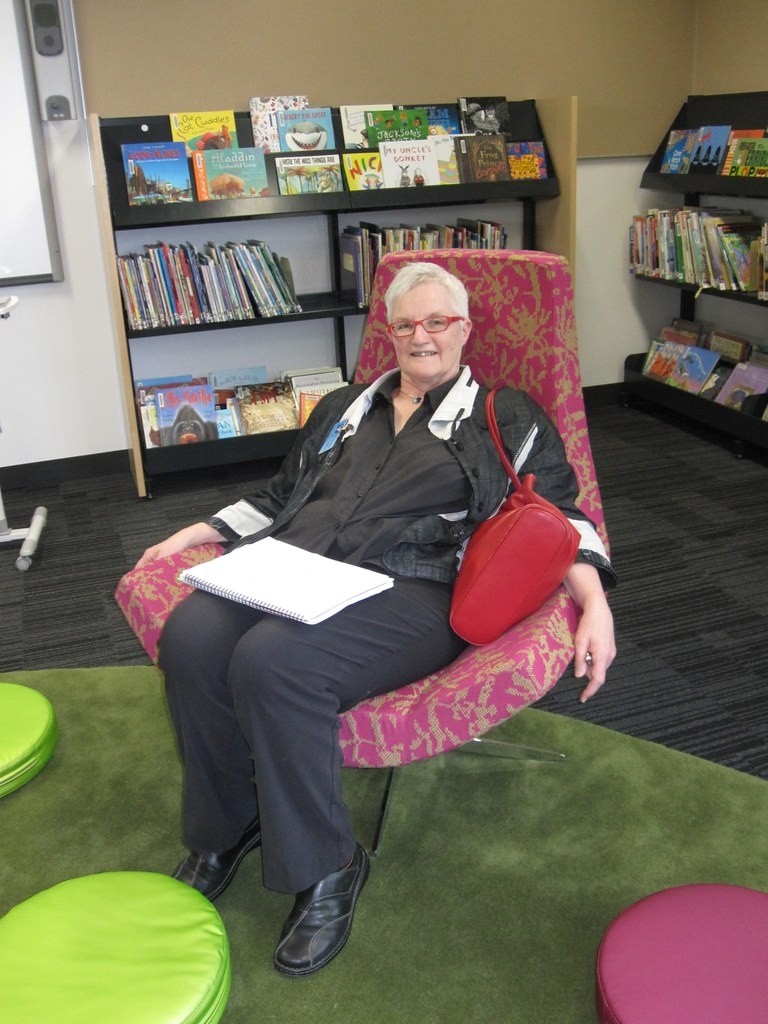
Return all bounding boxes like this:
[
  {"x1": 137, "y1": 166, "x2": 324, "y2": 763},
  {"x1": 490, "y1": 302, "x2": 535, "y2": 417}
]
[
  {"x1": 618, "y1": 89, "x2": 767, "y2": 465},
  {"x1": 89, "y1": 96, "x2": 583, "y2": 500}
]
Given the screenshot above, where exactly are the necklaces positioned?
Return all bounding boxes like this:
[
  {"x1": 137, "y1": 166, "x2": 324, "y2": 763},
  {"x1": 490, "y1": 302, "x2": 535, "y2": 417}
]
[{"x1": 398, "y1": 388, "x2": 424, "y2": 404}]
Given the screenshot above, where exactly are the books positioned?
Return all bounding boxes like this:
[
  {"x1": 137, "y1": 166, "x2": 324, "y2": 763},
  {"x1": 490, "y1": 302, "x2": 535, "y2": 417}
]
[
  {"x1": 632, "y1": 122, "x2": 768, "y2": 424},
  {"x1": 113, "y1": 95, "x2": 555, "y2": 446},
  {"x1": 176, "y1": 535, "x2": 397, "y2": 628}
]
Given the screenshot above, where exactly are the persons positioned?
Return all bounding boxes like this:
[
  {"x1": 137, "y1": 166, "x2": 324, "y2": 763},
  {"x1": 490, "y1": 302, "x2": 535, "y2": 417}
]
[{"x1": 131, "y1": 263, "x2": 621, "y2": 987}]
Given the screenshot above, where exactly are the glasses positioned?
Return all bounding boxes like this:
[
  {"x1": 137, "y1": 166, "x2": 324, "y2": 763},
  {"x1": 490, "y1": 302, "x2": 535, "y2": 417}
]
[{"x1": 389, "y1": 314, "x2": 465, "y2": 337}]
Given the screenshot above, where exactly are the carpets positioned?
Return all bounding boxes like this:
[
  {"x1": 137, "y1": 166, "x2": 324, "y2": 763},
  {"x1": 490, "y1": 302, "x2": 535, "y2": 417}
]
[{"x1": 1, "y1": 665, "x2": 768, "y2": 1021}]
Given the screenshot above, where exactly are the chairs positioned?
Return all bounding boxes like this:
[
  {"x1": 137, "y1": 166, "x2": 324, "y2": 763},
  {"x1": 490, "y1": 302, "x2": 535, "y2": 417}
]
[{"x1": 114, "y1": 247, "x2": 608, "y2": 863}]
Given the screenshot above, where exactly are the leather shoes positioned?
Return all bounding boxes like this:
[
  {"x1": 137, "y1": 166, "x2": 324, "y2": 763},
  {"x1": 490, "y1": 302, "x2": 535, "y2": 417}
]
[
  {"x1": 273, "y1": 841, "x2": 370, "y2": 977},
  {"x1": 171, "y1": 807, "x2": 261, "y2": 904}
]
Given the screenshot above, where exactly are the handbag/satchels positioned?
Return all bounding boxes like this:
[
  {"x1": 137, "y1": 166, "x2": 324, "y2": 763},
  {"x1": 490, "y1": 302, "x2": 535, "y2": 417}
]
[{"x1": 449, "y1": 473, "x2": 581, "y2": 647}]
